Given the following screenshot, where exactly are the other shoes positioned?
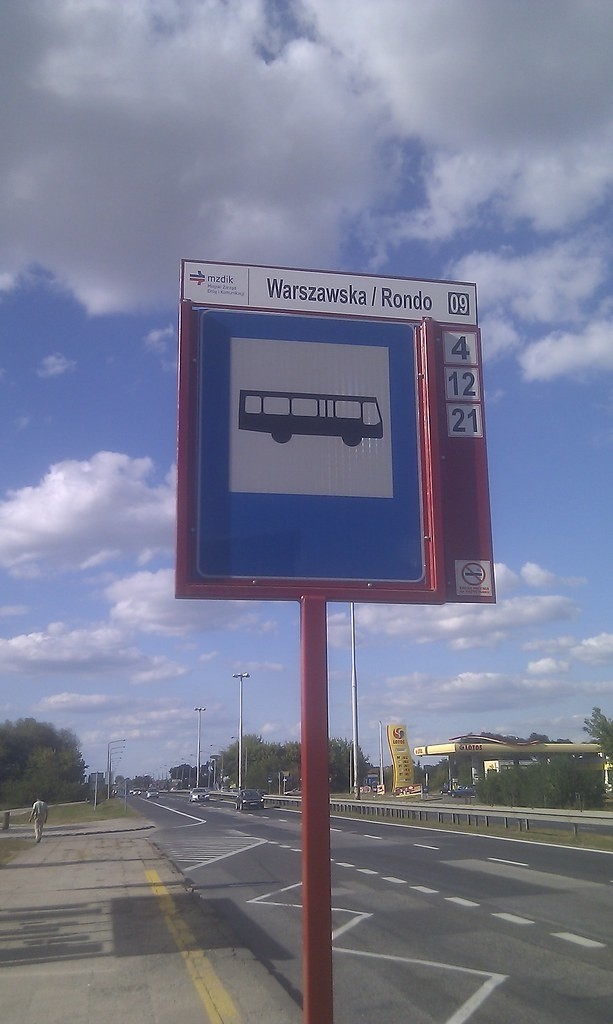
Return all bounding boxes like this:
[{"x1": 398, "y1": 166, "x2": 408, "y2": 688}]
[{"x1": 37, "y1": 837, "x2": 41, "y2": 843}]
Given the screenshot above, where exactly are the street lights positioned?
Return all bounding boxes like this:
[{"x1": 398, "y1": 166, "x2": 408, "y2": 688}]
[
  {"x1": 109, "y1": 745, "x2": 126, "y2": 799},
  {"x1": 232, "y1": 672, "x2": 251, "y2": 793},
  {"x1": 210, "y1": 744, "x2": 225, "y2": 783},
  {"x1": 107, "y1": 738, "x2": 126, "y2": 800},
  {"x1": 193, "y1": 707, "x2": 206, "y2": 788}
]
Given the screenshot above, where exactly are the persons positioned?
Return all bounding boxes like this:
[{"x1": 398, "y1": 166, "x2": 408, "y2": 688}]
[{"x1": 28, "y1": 795, "x2": 48, "y2": 844}]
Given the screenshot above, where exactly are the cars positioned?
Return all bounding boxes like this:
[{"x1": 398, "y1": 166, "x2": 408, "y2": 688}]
[
  {"x1": 255, "y1": 787, "x2": 268, "y2": 797},
  {"x1": 146, "y1": 787, "x2": 160, "y2": 799},
  {"x1": 129, "y1": 786, "x2": 142, "y2": 796},
  {"x1": 285, "y1": 788, "x2": 302, "y2": 796},
  {"x1": 422, "y1": 785, "x2": 431, "y2": 793},
  {"x1": 189, "y1": 788, "x2": 211, "y2": 802},
  {"x1": 235, "y1": 789, "x2": 264, "y2": 812},
  {"x1": 440, "y1": 783, "x2": 477, "y2": 798}
]
